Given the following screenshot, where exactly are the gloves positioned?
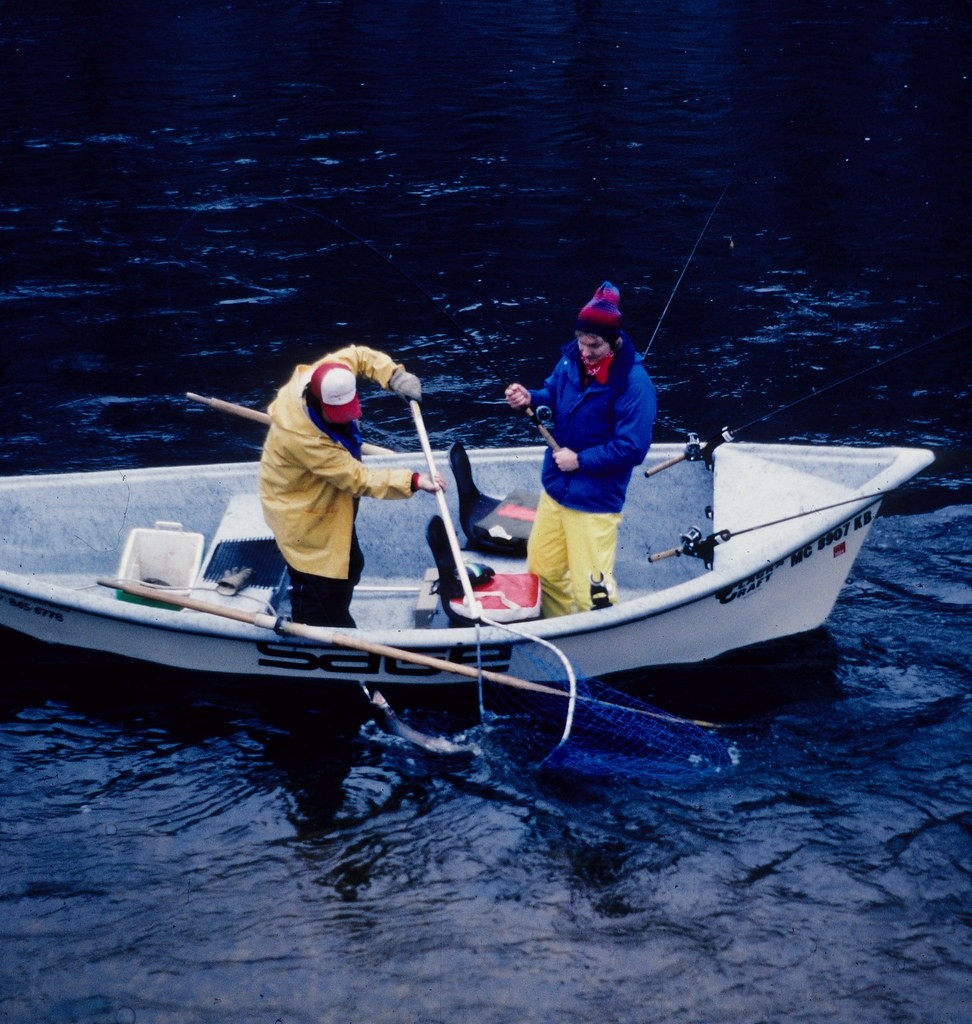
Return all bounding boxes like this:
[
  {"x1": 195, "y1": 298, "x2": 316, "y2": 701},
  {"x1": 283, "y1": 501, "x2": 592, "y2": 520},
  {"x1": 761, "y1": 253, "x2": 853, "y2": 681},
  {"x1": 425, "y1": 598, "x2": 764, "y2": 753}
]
[{"x1": 389, "y1": 365, "x2": 422, "y2": 406}]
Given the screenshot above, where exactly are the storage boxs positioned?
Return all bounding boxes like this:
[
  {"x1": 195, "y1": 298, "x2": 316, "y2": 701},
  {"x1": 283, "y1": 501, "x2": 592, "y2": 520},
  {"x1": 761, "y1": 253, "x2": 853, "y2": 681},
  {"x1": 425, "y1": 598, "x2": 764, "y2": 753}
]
[{"x1": 116, "y1": 521, "x2": 205, "y2": 612}]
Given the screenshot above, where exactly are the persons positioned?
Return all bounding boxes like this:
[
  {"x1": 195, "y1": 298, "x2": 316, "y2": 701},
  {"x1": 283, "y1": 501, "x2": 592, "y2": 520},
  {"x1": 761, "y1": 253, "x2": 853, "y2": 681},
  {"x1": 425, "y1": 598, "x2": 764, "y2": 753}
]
[
  {"x1": 504, "y1": 279, "x2": 656, "y2": 618},
  {"x1": 258, "y1": 343, "x2": 448, "y2": 627}
]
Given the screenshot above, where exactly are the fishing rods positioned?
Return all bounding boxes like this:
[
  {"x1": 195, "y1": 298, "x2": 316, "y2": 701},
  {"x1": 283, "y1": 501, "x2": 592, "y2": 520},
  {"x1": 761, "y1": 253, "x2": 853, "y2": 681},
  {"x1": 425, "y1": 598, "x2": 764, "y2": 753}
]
[
  {"x1": 642, "y1": 319, "x2": 972, "y2": 481},
  {"x1": 187, "y1": 195, "x2": 558, "y2": 451},
  {"x1": 647, "y1": 466, "x2": 970, "y2": 563}
]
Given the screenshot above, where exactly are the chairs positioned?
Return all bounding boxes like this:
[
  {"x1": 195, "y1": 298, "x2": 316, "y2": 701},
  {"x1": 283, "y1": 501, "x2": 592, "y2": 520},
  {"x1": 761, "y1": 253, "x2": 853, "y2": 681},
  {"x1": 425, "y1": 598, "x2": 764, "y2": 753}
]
[
  {"x1": 425, "y1": 516, "x2": 543, "y2": 629},
  {"x1": 448, "y1": 441, "x2": 529, "y2": 555}
]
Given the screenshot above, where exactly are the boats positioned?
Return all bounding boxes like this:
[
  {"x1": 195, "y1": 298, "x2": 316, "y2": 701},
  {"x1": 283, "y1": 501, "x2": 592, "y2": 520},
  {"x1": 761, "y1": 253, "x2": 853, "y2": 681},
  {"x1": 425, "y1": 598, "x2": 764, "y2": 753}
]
[{"x1": 2, "y1": 439, "x2": 934, "y2": 737}]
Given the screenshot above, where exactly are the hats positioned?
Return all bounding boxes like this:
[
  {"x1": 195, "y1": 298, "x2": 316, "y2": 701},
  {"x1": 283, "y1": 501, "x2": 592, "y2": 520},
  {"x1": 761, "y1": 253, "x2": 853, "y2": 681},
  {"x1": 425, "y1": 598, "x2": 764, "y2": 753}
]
[
  {"x1": 310, "y1": 362, "x2": 362, "y2": 423},
  {"x1": 574, "y1": 281, "x2": 621, "y2": 342}
]
